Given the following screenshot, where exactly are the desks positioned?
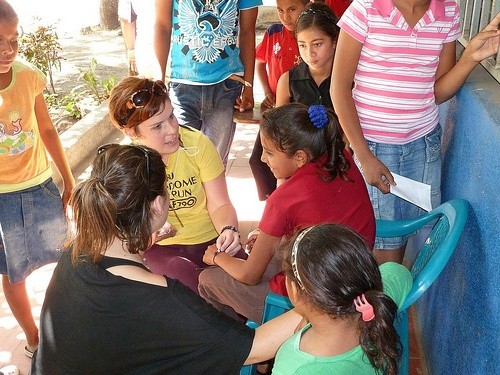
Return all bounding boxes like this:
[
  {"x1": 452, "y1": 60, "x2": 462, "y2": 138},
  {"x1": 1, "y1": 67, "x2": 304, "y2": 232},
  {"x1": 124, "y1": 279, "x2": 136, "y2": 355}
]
[{"x1": 233, "y1": 101, "x2": 266, "y2": 125}]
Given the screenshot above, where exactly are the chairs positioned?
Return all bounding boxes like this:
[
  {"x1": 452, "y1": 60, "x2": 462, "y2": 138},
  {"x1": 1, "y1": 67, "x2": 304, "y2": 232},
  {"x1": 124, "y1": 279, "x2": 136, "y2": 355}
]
[
  {"x1": 259, "y1": 198, "x2": 470, "y2": 375},
  {"x1": 376, "y1": 261, "x2": 412, "y2": 311}
]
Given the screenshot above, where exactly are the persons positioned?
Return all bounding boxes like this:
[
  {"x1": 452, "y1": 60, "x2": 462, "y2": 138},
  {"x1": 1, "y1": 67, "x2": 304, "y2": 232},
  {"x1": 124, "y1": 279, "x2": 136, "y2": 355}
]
[
  {"x1": 0, "y1": 0, "x2": 76, "y2": 359},
  {"x1": 272, "y1": 223, "x2": 413, "y2": 375},
  {"x1": 154, "y1": 0, "x2": 263, "y2": 170},
  {"x1": 249, "y1": 0, "x2": 310, "y2": 201},
  {"x1": 275, "y1": 3, "x2": 355, "y2": 108},
  {"x1": 330, "y1": 0, "x2": 500, "y2": 265},
  {"x1": 199, "y1": 103, "x2": 377, "y2": 326},
  {"x1": 30, "y1": 143, "x2": 310, "y2": 375},
  {"x1": 118, "y1": 0, "x2": 163, "y2": 82},
  {"x1": 109, "y1": 77, "x2": 249, "y2": 296}
]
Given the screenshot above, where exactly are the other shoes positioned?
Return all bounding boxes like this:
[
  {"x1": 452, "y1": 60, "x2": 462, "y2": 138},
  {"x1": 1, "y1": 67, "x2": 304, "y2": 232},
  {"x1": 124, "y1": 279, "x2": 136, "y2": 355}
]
[
  {"x1": 0, "y1": 350, "x2": 20, "y2": 375},
  {"x1": 128, "y1": 60, "x2": 139, "y2": 76},
  {"x1": 23, "y1": 345, "x2": 34, "y2": 358}
]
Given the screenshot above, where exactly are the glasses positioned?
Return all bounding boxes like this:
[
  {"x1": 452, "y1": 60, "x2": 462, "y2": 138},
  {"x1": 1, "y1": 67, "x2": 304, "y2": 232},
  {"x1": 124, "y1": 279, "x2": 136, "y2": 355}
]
[
  {"x1": 96, "y1": 143, "x2": 153, "y2": 195},
  {"x1": 119, "y1": 79, "x2": 167, "y2": 127}
]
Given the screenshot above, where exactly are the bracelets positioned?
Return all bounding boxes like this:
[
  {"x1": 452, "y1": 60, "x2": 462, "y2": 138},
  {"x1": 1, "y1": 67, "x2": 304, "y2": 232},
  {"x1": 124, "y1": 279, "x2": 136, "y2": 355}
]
[
  {"x1": 212, "y1": 251, "x2": 220, "y2": 265},
  {"x1": 221, "y1": 225, "x2": 241, "y2": 239}
]
